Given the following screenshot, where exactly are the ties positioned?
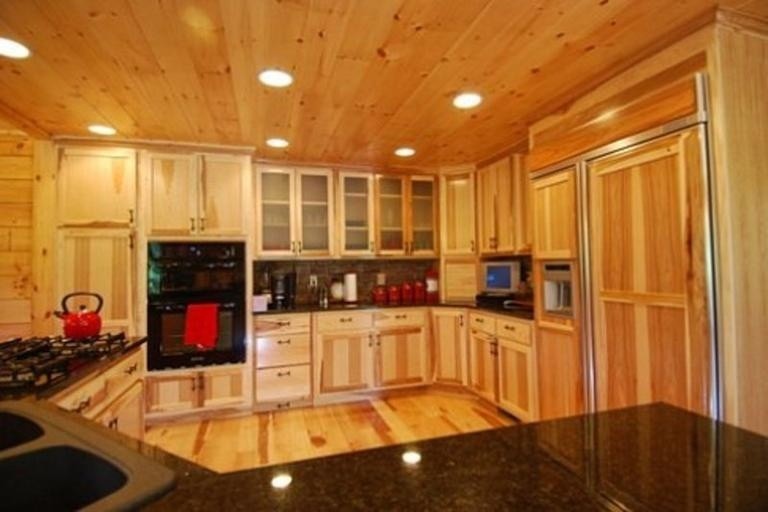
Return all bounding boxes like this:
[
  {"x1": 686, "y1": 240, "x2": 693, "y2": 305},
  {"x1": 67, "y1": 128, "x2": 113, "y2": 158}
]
[{"x1": 479, "y1": 260, "x2": 520, "y2": 295}]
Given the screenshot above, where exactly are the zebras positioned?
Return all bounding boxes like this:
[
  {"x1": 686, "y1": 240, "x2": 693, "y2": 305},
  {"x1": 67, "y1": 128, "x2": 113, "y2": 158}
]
[{"x1": 144, "y1": 240, "x2": 247, "y2": 370}]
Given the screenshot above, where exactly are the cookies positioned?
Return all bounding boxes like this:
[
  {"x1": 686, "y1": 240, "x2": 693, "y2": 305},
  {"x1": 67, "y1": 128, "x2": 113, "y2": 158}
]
[{"x1": 539, "y1": 259, "x2": 576, "y2": 323}]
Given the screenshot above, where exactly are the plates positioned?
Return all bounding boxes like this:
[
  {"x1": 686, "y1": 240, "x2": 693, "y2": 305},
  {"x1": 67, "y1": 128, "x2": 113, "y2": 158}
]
[
  {"x1": 376, "y1": 172, "x2": 439, "y2": 259},
  {"x1": 532, "y1": 163, "x2": 579, "y2": 261},
  {"x1": 438, "y1": 168, "x2": 481, "y2": 305},
  {"x1": 255, "y1": 162, "x2": 337, "y2": 260},
  {"x1": 532, "y1": 319, "x2": 583, "y2": 425},
  {"x1": 146, "y1": 364, "x2": 254, "y2": 427},
  {"x1": 49, "y1": 141, "x2": 138, "y2": 335},
  {"x1": 254, "y1": 310, "x2": 311, "y2": 413},
  {"x1": 138, "y1": 143, "x2": 255, "y2": 237},
  {"x1": 482, "y1": 154, "x2": 531, "y2": 256},
  {"x1": 338, "y1": 168, "x2": 375, "y2": 259},
  {"x1": 427, "y1": 304, "x2": 466, "y2": 387},
  {"x1": 465, "y1": 307, "x2": 531, "y2": 426},
  {"x1": 311, "y1": 307, "x2": 427, "y2": 402}
]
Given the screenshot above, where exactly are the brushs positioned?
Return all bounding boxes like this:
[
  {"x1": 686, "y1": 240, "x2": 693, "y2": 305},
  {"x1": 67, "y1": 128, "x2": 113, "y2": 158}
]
[{"x1": 285, "y1": 271, "x2": 298, "y2": 312}]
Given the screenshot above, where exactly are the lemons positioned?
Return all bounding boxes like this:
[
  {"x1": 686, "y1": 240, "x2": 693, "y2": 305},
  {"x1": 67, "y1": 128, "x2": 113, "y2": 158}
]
[{"x1": 51, "y1": 290, "x2": 105, "y2": 337}]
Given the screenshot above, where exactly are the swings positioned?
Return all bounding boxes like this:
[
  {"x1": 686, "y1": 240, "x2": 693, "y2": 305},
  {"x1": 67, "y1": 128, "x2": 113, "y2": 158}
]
[
  {"x1": 254, "y1": 261, "x2": 272, "y2": 311},
  {"x1": 271, "y1": 272, "x2": 286, "y2": 310}
]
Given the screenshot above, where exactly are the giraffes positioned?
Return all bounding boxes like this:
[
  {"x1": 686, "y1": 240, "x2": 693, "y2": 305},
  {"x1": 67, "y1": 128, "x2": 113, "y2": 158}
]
[{"x1": 0, "y1": 332, "x2": 128, "y2": 391}]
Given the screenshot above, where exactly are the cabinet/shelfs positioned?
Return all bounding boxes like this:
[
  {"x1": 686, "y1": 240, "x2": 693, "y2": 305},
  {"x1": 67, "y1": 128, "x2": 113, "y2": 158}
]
[
  {"x1": 370, "y1": 266, "x2": 438, "y2": 304},
  {"x1": 343, "y1": 270, "x2": 359, "y2": 303}
]
